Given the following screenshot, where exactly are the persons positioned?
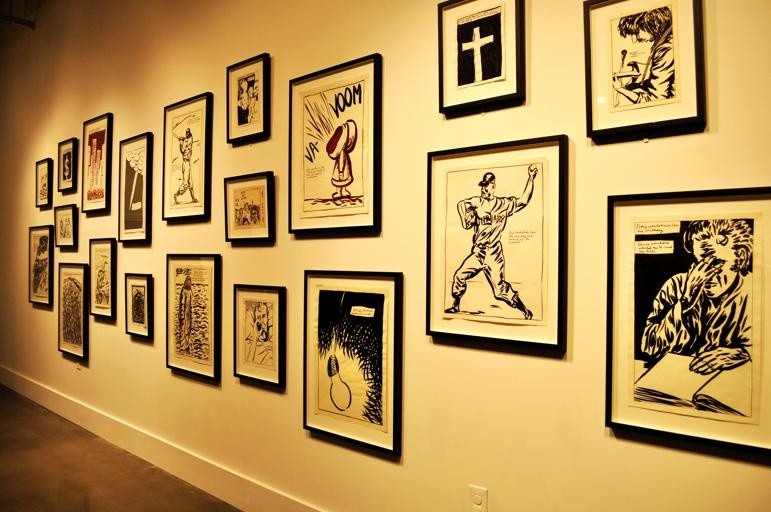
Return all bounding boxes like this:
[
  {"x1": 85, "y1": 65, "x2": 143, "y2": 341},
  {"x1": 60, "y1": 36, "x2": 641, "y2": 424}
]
[
  {"x1": 179, "y1": 272, "x2": 193, "y2": 355},
  {"x1": 639, "y1": 221, "x2": 753, "y2": 375},
  {"x1": 243, "y1": 298, "x2": 273, "y2": 370},
  {"x1": 231, "y1": 198, "x2": 261, "y2": 225},
  {"x1": 444, "y1": 168, "x2": 539, "y2": 319},
  {"x1": 174, "y1": 128, "x2": 197, "y2": 205},
  {"x1": 611, "y1": 6, "x2": 677, "y2": 102}
]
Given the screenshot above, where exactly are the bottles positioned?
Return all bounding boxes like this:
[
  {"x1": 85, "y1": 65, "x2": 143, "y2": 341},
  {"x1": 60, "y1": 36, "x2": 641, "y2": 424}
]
[{"x1": 478, "y1": 172, "x2": 497, "y2": 187}]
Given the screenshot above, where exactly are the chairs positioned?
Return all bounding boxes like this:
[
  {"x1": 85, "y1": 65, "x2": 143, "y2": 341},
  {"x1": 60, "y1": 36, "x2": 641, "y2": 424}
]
[
  {"x1": 444, "y1": 299, "x2": 460, "y2": 312},
  {"x1": 514, "y1": 298, "x2": 533, "y2": 320}
]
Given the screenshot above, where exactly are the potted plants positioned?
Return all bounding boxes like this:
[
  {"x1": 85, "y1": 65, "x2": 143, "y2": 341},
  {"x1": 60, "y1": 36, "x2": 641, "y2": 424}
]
[
  {"x1": 221, "y1": 169, "x2": 277, "y2": 247},
  {"x1": 232, "y1": 284, "x2": 288, "y2": 394},
  {"x1": 288, "y1": 53, "x2": 384, "y2": 236},
  {"x1": 605, "y1": 185, "x2": 771, "y2": 465},
  {"x1": 226, "y1": 52, "x2": 273, "y2": 146},
  {"x1": 436, "y1": 1, "x2": 527, "y2": 120},
  {"x1": 426, "y1": 135, "x2": 570, "y2": 362},
  {"x1": 303, "y1": 269, "x2": 405, "y2": 460},
  {"x1": 582, "y1": 0, "x2": 708, "y2": 145}
]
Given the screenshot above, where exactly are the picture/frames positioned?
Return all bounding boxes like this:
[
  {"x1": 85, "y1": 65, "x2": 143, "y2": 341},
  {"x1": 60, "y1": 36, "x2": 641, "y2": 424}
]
[
  {"x1": 221, "y1": 169, "x2": 277, "y2": 247},
  {"x1": 426, "y1": 135, "x2": 570, "y2": 362},
  {"x1": 87, "y1": 237, "x2": 117, "y2": 319},
  {"x1": 82, "y1": 112, "x2": 113, "y2": 212},
  {"x1": 436, "y1": 1, "x2": 527, "y2": 120},
  {"x1": 582, "y1": 0, "x2": 708, "y2": 145},
  {"x1": 58, "y1": 137, "x2": 78, "y2": 191},
  {"x1": 288, "y1": 53, "x2": 384, "y2": 236},
  {"x1": 303, "y1": 269, "x2": 405, "y2": 460},
  {"x1": 29, "y1": 224, "x2": 55, "y2": 307},
  {"x1": 226, "y1": 52, "x2": 273, "y2": 146},
  {"x1": 58, "y1": 262, "x2": 90, "y2": 359},
  {"x1": 166, "y1": 253, "x2": 220, "y2": 380},
  {"x1": 232, "y1": 284, "x2": 288, "y2": 394},
  {"x1": 119, "y1": 132, "x2": 152, "y2": 242},
  {"x1": 163, "y1": 93, "x2": 212, "y2": 221},
  {"x1": 35, "y1": 157, "x2": 51, "y2": 207},
  {"x1": 53, "y1": 204, "x2": 76, "y2": 247},
  {"x1": 124, "y1": 272, "x2": 154, "y2": 340},
  {"x1": 605, "y1": 185, "x2": 771, "y2": 465}
]
